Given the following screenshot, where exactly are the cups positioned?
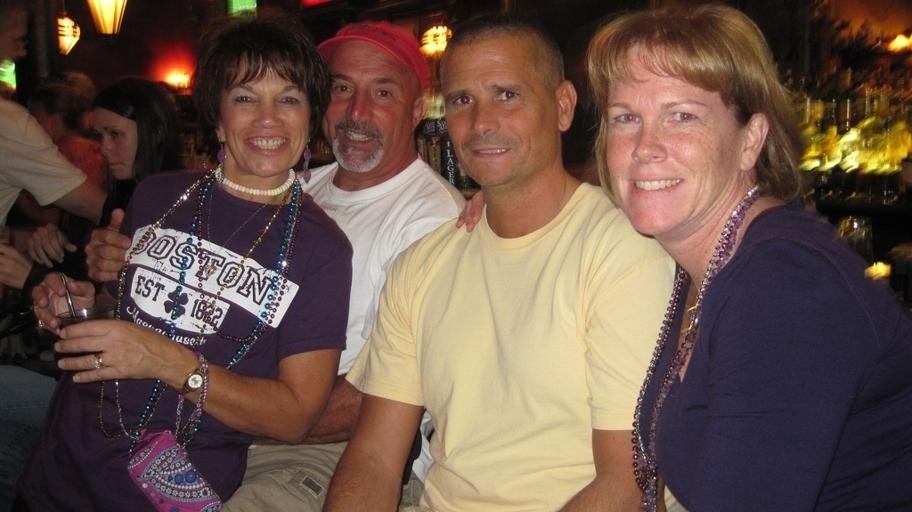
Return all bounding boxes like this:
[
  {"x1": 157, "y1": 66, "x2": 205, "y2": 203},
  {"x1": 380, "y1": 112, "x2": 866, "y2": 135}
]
[{"x1": 55, "y1": 306, "x2": 115, "y2": 375}]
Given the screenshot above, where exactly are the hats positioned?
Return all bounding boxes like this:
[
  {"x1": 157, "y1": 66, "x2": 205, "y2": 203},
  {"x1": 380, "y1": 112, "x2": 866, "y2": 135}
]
[{"x1": 315, "y1": 21, "x2": 431, "y2": 90}]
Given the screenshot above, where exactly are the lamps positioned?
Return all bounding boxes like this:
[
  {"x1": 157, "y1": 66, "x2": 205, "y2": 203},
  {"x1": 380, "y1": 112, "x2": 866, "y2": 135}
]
[{"x1": 69, "y1": 0, "x2": 128, "y2": 46}]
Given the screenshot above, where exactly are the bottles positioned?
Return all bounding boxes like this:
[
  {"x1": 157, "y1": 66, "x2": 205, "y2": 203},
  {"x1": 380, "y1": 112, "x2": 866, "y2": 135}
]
[{"x1": 782, "y1": 57, "x2": 912, "y2": 139}]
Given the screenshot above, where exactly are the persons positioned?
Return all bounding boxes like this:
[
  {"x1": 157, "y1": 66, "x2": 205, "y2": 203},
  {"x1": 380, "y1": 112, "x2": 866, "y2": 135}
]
[{"x1": 1, "y1": 0, "x2": 912, "y2": 512}]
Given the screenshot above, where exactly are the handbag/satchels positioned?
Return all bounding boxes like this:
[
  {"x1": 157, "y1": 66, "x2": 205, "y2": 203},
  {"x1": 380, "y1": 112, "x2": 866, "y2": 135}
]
[{"x1": 125, "y1": 431, "x2": 223, "y2": 511}]
